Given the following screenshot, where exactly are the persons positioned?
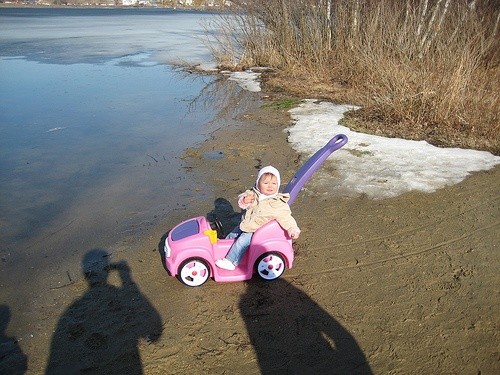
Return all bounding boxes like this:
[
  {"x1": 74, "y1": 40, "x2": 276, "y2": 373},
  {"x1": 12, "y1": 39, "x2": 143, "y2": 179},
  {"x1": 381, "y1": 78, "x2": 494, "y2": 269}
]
[{"x1": 214, "y1": 166, "x2": 302, "y2": 270}]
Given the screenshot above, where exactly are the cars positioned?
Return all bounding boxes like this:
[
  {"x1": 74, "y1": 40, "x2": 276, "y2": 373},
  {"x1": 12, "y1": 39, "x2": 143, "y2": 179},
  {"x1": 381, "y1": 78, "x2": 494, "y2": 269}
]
[{"x1": 162, "y1": 134, "x2": 350, "y2": 288}]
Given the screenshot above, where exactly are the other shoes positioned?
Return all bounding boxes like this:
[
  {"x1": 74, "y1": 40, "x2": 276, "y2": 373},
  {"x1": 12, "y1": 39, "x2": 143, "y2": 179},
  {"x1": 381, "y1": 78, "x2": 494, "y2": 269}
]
[{"x1": 215, "y1": 258, "x2": 235, "y2": 270}]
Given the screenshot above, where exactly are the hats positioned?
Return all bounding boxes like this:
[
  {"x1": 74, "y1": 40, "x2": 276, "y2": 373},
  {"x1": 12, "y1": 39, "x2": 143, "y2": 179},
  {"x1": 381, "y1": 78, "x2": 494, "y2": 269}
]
[{"x1": 256, "y1": 165, "x2": 281, "y2": 197}]
